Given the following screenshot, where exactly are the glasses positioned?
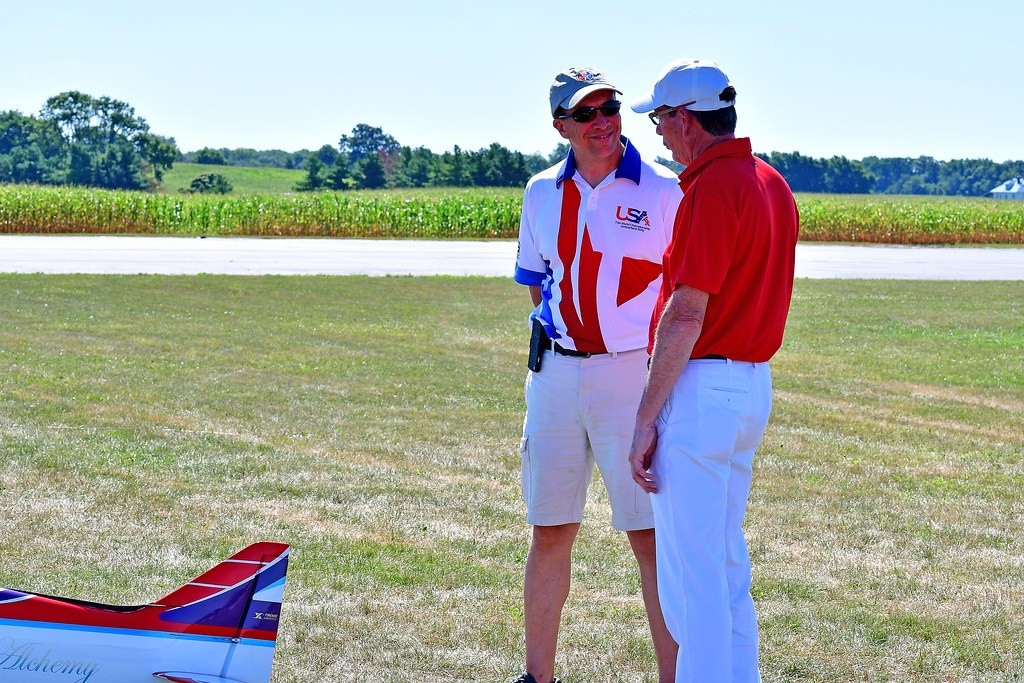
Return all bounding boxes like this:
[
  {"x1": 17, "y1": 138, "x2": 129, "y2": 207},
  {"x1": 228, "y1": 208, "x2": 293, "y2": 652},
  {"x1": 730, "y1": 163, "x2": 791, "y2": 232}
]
[
  {"x1": 558, "y1": 100, "x2": 621, "y2": 123},
  {"x1": 649, "y1": 101, "x2": 696, "y2": 125}
]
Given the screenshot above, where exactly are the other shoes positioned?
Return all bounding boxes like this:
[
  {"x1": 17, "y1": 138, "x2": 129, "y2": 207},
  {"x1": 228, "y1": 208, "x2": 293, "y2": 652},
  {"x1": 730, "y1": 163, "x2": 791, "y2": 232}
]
[{"x1": 512, "y1": 672, "x2": 562, "y2": 683}]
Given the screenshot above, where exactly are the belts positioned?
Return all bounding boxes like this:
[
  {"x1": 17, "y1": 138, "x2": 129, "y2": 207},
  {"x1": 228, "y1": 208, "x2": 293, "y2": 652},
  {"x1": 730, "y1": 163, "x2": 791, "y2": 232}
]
[
  {"x1": 546, "y1": 340, "x2": 608, "y2": 356},
  {"x1": 689, "y1": 354, "x2": 727, "y2": 360}
]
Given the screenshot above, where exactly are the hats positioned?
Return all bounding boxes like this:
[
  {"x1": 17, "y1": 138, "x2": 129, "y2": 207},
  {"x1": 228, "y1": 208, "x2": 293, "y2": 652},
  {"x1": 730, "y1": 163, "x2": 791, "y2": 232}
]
[
  {"x1": 630, "y1": 59, "x2": 736, "y2": 113},
  {"x1": 550, "y1": 67, "x2": 623, "y2": 117}
]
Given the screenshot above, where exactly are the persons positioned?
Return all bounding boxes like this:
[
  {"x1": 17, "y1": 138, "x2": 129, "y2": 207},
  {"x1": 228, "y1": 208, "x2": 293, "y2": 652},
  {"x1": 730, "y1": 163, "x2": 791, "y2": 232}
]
[
  {"x1": 628, "y1": 61, "x2": 800, "y2": 683},
  {"x1": 513, "y1": 66, "x2": 684, "y2": 683}
]
